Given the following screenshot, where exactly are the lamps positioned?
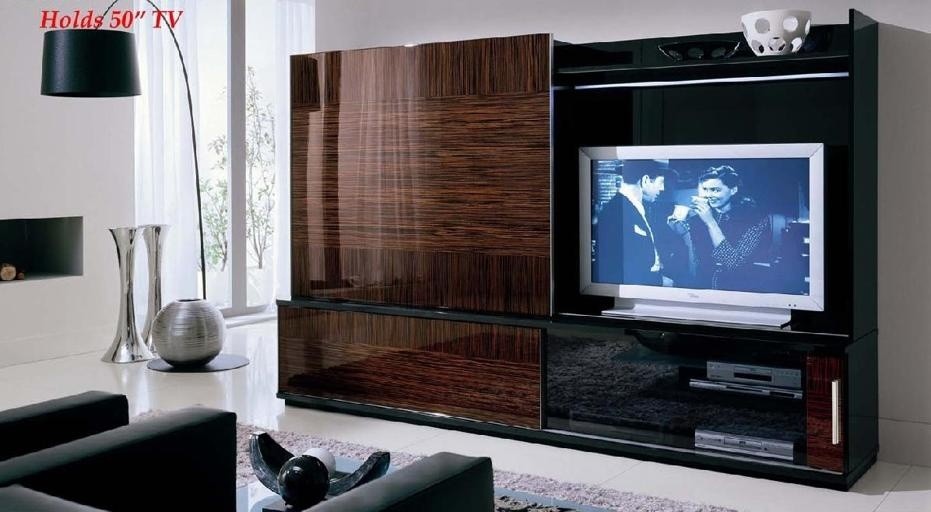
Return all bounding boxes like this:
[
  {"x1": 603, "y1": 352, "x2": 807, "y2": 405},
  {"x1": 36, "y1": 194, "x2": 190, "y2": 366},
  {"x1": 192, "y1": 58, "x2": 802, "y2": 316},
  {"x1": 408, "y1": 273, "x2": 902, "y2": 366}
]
[{"x1": 41, "y1": 0, "x2": 254, "y2": 371}]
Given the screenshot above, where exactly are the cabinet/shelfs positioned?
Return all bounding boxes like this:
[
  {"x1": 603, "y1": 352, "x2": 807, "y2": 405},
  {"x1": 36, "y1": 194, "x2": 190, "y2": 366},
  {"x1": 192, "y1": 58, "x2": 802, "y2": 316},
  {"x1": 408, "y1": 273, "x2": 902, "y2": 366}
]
[
  {"x1": 543, "y1": 321, "x2": 882, "y2": 491},
  {"x1": 276, "y1": 32, "x2": 547, "y2": 434}
]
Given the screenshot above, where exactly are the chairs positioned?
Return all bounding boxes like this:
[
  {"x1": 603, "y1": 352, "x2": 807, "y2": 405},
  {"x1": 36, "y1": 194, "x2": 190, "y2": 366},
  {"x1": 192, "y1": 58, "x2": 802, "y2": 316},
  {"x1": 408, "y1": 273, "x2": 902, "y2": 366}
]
[{"x1": 0, "y1": 403, "x2": 494, "y2": 512}]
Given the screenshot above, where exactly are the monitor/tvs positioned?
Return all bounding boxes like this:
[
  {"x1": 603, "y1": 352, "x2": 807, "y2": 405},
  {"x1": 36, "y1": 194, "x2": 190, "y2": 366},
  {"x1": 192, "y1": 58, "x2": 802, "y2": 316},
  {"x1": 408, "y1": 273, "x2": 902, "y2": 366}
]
[{"x1": 578, "y1": 142, "x2": 825, "y2": 330}]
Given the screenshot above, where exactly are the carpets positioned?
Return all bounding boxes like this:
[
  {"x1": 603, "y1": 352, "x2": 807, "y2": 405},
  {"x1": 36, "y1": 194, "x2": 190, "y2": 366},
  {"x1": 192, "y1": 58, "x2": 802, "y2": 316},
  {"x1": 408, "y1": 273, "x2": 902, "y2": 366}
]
[{"x1": 128, "y1": 403, "x2": 739, "y2": 512}]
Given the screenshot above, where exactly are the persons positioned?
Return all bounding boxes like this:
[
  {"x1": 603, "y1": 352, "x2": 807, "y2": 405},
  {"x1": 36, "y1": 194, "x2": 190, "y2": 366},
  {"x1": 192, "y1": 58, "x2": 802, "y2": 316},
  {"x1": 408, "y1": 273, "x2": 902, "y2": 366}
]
[
  {"x1": 598, "y1": 160, "x2": 674, "y2": 287},
  {"x1": 667, "y1": 166, "x2": 772, "y2": 290}
]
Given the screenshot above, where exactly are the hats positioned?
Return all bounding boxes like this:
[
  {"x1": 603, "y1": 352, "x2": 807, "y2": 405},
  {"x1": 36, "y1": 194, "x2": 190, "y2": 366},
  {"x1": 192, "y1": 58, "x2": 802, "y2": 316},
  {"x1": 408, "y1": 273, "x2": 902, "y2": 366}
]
[{"x1": 615, "y1": 159, "x2": 678, "y2": 174}]
